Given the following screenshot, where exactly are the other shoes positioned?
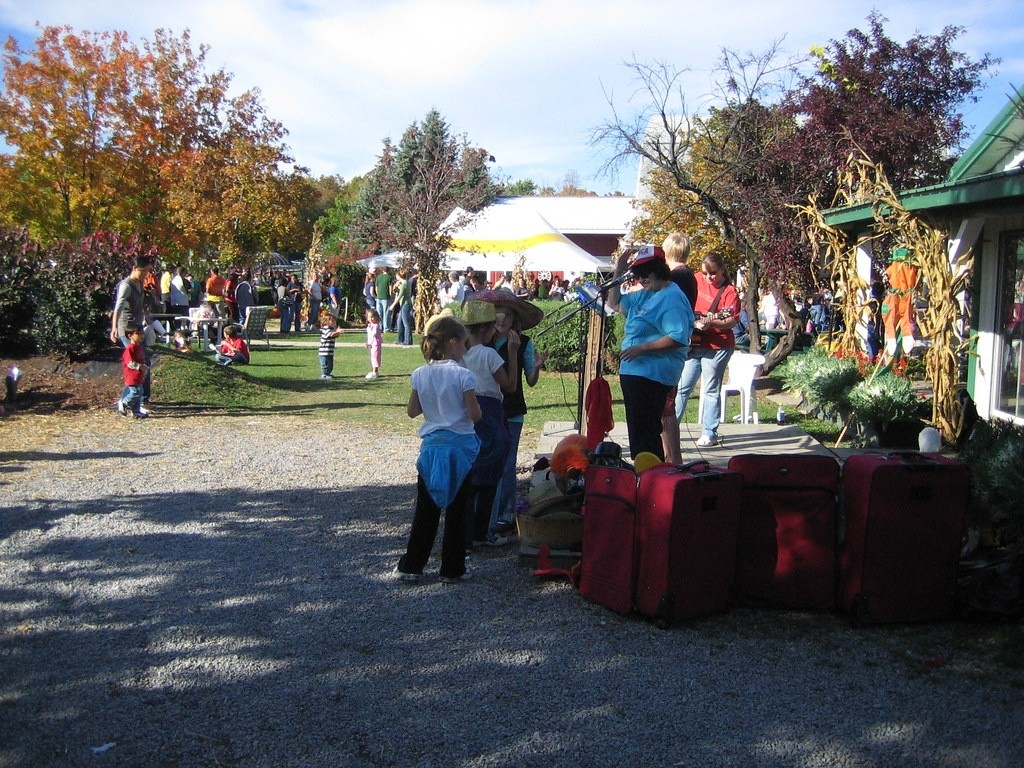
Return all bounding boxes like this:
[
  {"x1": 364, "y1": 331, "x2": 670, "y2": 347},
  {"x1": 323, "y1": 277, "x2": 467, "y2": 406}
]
[
  {"x1": 393, "y1": 565, "x2": 421, "y2": 582},
  {"x1": 473, "y1": 534, "x2": 508, "y2": 547},
  {"x1": 438, "y1": 576, "x2": 459, "y2": 583},
  {"x1": 116, "y1": 398, "x2": 127, "y2": 416},
  {"x1": 490, "y1": 521, "x2": 516, "y2": 534},
  {"x1": 140, "y1": 405, "x2": 156, "y2": 411},
  {"x1": 133, "y1": 413, "x2": 148, "y2": 419}
]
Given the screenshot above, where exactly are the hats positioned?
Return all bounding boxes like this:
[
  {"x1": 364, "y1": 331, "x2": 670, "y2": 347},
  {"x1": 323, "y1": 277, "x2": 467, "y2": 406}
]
[
  {"x1": 632, "y1": 246, "x2": 666, "y2": 262},
  {"x1": 593, "y1": 442, "x2": 625, "y2": 466},
  {"x1": 424, "y1": 285, "x2": 547, "y2": 336}
]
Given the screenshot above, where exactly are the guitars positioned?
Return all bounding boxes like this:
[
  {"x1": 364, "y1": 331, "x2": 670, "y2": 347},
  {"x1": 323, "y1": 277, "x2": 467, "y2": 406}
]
[{"x1": 689, "y1": 304, "x2": 734, "y2": 353}]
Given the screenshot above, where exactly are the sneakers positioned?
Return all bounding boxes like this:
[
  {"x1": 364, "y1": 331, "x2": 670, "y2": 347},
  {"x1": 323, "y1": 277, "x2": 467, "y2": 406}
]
[
  {"x1": 319, "y1": 372, "x2": 335, "y2": 379},
  {"x1": 695, "y1": 434, "x2": 717, "y2": 450},
  {"x1": 366, "y1": 371, "x2": 380, "y2": 380}
]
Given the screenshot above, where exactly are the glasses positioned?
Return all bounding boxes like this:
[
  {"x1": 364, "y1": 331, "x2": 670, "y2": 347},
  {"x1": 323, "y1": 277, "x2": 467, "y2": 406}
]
[
  {"x1": 628, "y1": 271, "x2": 655, "y2": 279},
  {"x1": 701, "y1": 270, "x2": 721, "y2": 276}
]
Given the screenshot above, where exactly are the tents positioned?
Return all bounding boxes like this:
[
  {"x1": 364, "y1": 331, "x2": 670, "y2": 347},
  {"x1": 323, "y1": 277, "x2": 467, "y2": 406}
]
[{"x1": 357, "y1": 204, "x2": 616, "y2": 288}]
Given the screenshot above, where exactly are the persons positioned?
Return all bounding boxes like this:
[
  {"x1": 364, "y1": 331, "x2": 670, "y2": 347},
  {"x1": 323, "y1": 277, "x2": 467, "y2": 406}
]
[
  {"x1": 215, "y1": 325, "x2": 249, "y2": 365},
  {"x1": 735, "y1": 256, "x2": 846, "y2": 356},
  {"x1": 1015, "y1": 278, "x2": 1024, "y2": 323},
  {"x1": 398, "y1": 316, "x2": 481, "y2": 582},
  {"x1": 355, "y1": 268, "x2": 592, "y2": 346},
  {"x1": 867, "y1": 283, "x2": 886, "y2": 363},
  {"x1": 366, "y1": 310, "x2": 384, "y2": 381},
  {"x1": 319, "y1": 314, "x2": 344, "y2": 380},
  {"x1": 455, "y1": 287, "x2": 551, "y2": 547},
  {"x1": 607, "y1": 233, "x2": 697, "y2": 465},
  {"x1": 139, "y1": 261, "x2": 344, "y2": 335},
  {"x1": 674, "y1": 233, "x2": 741, "y2": 445},
  {"x1": 111, "y1": 257, "x2": 156, "y2": 410},
  {"x1": 116, "y1": 323, "x2": 149, "y2": 418}
]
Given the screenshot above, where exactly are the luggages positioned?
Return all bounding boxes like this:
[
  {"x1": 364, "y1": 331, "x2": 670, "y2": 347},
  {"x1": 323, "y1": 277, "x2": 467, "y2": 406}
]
[
  {"x1": 580, "y1": 462, "x2": 727, "y2": 630},
  {"x1": 723, "y1": 454, "x2": 844, "y2": 612},
  {"x1": 841, "y1": 450, "x2": 978, "y2": 627}
]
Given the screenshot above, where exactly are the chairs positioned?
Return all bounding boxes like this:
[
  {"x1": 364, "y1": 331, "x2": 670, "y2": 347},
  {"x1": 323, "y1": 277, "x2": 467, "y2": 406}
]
[
  {"x1": 237, "y1": 305, "x2": 271, "y2": 352},
  {"x1": 721, "y1": 353, "x2": 767, "y2": 425}
]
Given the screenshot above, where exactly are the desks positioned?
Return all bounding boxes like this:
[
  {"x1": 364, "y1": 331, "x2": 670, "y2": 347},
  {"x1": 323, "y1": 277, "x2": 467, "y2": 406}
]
[
  {"x1": 149, "y1": 313, "x2": 181, "y2": 336},
  {"x1": 175, "y1": 316, "x2": 227, "y2": 353}
]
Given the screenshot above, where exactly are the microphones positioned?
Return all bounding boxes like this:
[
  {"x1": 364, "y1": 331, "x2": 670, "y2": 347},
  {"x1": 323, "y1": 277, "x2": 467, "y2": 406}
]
[{"x1": 598, "y1": 277, "x2": 625, "y2": 293}]
